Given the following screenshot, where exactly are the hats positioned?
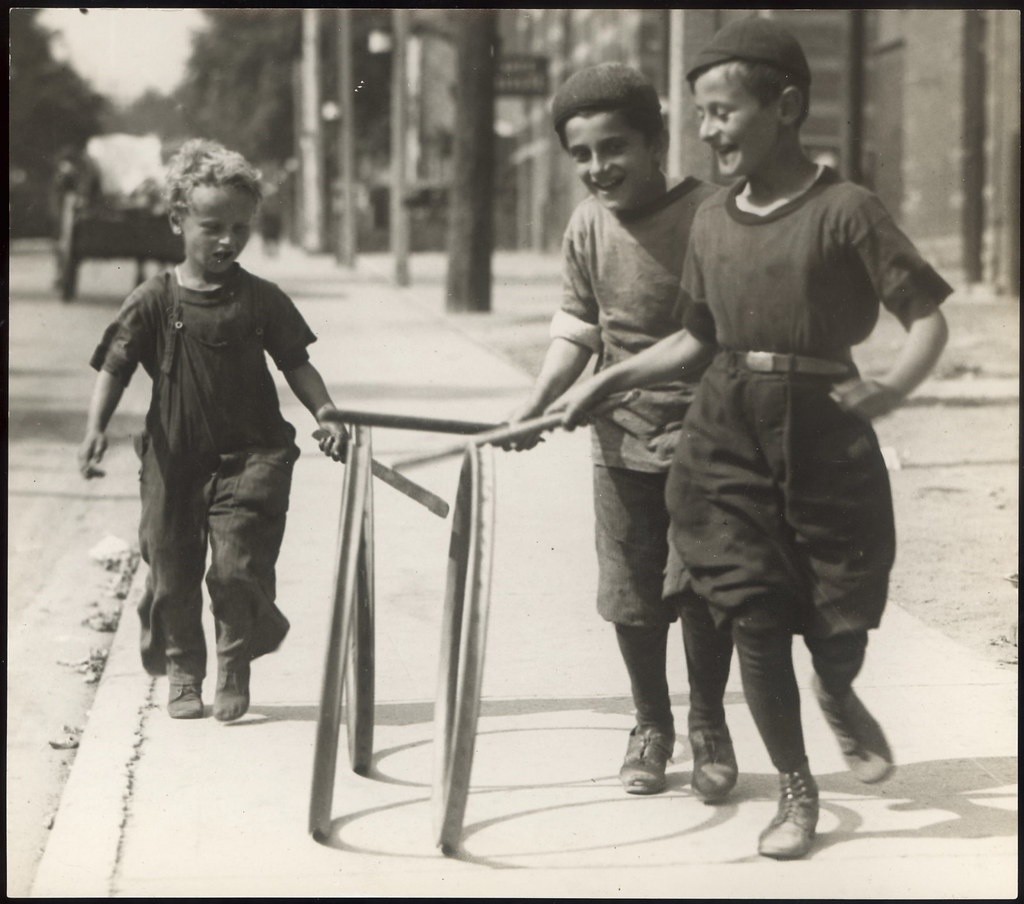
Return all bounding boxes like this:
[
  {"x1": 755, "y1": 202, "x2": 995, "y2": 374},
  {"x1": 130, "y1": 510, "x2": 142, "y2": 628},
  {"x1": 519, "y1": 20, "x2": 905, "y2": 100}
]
[
  {"x1": 686, "y1": 15, "x2": 811, "y2": 86},
  {"x1": 551, "y1": 63, "x2": 662, "y2": 132}
]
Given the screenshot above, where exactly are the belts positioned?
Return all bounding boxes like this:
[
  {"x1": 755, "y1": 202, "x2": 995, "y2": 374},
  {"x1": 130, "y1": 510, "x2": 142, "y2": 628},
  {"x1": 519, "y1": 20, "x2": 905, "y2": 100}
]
[{"x1": 734, "y1": 352, "x2": 848, "y2": 375}]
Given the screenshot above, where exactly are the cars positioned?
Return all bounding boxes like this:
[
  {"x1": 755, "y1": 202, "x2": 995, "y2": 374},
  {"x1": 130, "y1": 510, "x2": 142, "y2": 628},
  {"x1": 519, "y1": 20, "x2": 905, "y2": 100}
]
[{"x1": 42, "y1": 126, "x2": 207, "y2": 298}]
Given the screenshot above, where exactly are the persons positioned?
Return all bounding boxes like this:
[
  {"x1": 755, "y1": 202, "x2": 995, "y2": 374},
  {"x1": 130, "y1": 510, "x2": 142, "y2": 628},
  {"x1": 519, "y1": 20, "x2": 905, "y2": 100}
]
[
  {"x1": 545, "y1": 13, "x2": 961, "y2": 863},
  {"x1": 78, "y1": 138, "x2": 350, "y2": 719},
  {"x1": 491, "y1": 59, "x2": 742, "y2": 805}
]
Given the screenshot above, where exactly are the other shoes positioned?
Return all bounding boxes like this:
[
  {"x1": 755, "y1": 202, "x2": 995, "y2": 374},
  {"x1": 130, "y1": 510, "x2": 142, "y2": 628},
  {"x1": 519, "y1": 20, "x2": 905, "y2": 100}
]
[
  {"x1": 212, "y1": 659, "x2": 250, "y2": 721},
  {"x1": 688, "y1": 722, "x2": 738, "y2": 804},
  {"x1": 758, "y1": 761, "x2": 819, "y2": 858},
  {"x1": 619, "y1": 721, "x2": 675, "y2": 793},
  {"x1": 167, "y1": 682, "x2": 203, "y2": 718},
  {"x1": 811, "y1": 674, "x2": 892, "y2": 783}
]
